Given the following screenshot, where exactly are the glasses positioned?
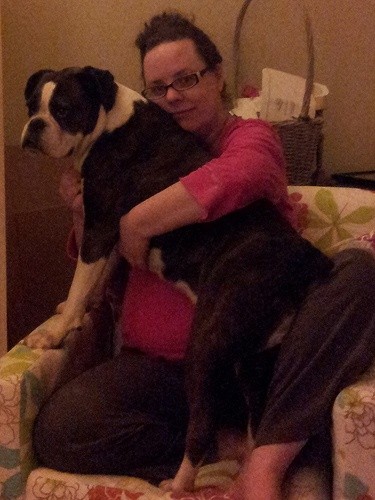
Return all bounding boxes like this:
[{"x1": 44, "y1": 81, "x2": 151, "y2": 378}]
[{"x1": 141, "y1": 63, "x2": 214, "y2": 100}]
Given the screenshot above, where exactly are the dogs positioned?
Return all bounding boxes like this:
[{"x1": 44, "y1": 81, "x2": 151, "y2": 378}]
[{"x1": 20, "y1": 64, "x2": 334, "y2": 493}]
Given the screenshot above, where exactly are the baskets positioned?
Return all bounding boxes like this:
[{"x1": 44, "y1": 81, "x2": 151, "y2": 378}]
[{"x1": 224, "y1": 0, "x2": 323, "y2": 184}]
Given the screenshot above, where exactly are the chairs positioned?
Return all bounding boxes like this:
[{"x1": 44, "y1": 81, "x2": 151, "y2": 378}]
[{"x1": 0, "y1": 185, "x2": 375, "y2": 500}]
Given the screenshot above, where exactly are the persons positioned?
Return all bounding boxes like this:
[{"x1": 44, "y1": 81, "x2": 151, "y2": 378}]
[{"x1": 32, "y1": 13, "x2": 374, "y2": 499}]
